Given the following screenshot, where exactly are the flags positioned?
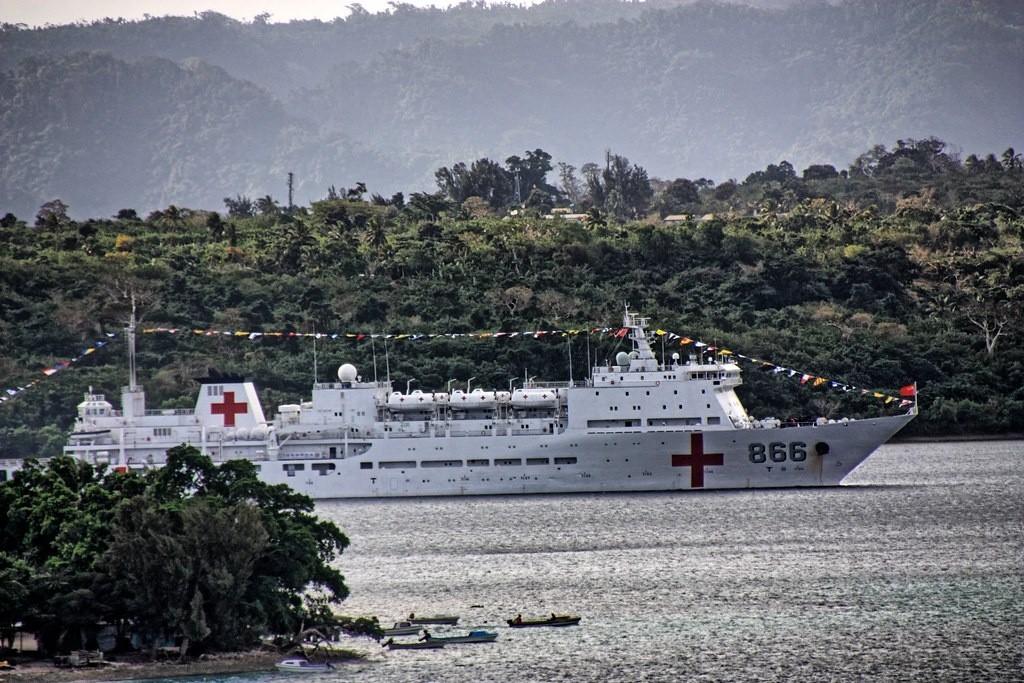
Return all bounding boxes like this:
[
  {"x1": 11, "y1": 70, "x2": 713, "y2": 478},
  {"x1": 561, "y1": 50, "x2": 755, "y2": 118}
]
[{"x1": 900, "y1": 384, "x2": 915, "y2": 396}]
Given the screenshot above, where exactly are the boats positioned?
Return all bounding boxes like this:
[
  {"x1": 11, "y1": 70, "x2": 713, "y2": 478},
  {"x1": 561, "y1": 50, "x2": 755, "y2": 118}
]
[
  {"x1": 418, "y1": 630, "x2": 498, "y2": 643},
  {"x1": 275, "y1": 660, "x2": 334, "y2": 673},
  {"x1": 382, "y1": 638, "x2": 447, "y2": 650},
  {"x1": 406, "y1": 613, "x2": 460, "y2": 625},
  {"x1": 0, "y1": 297, "x2": 918, "y2": 499},
  {"x1": 506, "y1": 615, "x2": 581, "y2": 628},
  {"x1": 382, "y1": 622, "x2": 423, "y2": 636}
]
